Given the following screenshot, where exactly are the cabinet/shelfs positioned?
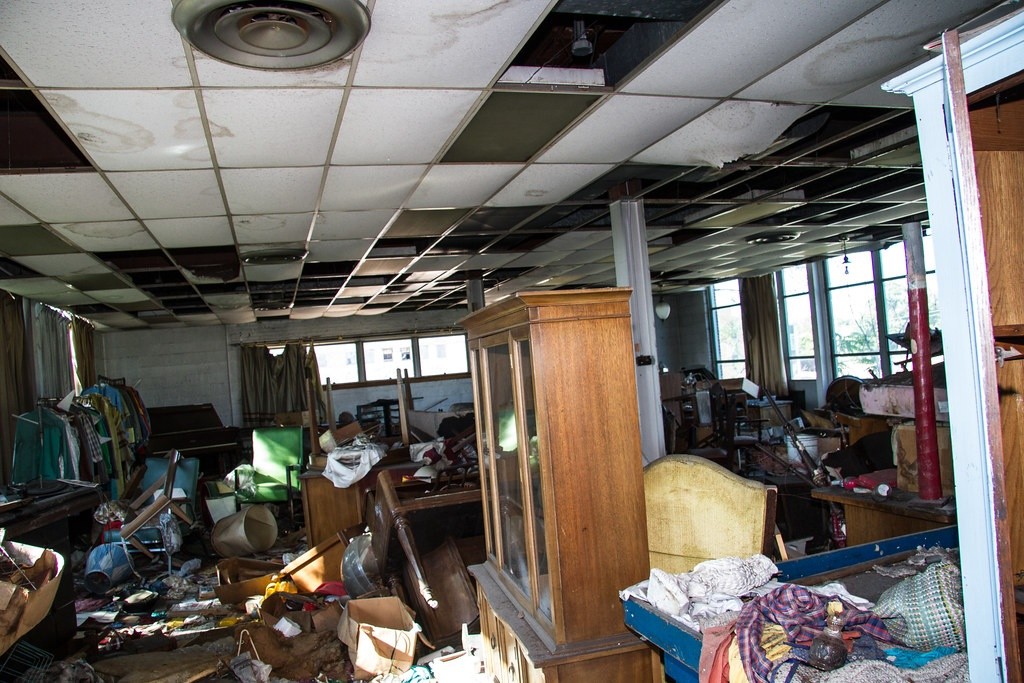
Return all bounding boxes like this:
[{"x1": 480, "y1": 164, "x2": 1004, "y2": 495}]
[
  {"x1": 467, "y1": 564, "x2": 662, "y2": 683},
  {"x1": 454, "y1": 287, "x2": 651, "y2": 653},
  {"x1": 883, "y1": 21, "x2": 1024, "y2": 683}
]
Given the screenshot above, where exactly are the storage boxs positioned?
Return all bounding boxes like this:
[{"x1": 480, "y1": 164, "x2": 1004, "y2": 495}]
[
  {"x1": 337, "y1": 596, "x2": 436, "y2": 681},
  {"x1": 0, "y1": 542, "x2": 65, "y2": 656},
  {"x1": 275, "y1": 410, "x2": 321, "y2": 428}
]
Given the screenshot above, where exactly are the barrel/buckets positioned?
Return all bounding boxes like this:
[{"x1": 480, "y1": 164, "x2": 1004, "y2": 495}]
[{"x1": 786, "y1": 433, "x2": 819, "y2": 465}]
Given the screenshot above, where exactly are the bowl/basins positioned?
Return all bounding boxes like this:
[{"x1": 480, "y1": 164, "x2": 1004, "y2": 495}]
[{"x1": 763, "y1": 395, "x2": 776, "y2": 403}]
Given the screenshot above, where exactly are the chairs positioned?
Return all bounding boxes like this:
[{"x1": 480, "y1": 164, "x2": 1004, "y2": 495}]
[
  {"x1": 680, "y1": 394, "x2": 736, "y2": 472},
  {"x1": 100, "y1": 456, "x2": 204, "y2": 575},
  {"x1": 235, "y1": 425, "x2": 307, "y2": 531},
  {"x1": 707, "y1": 383, "x2": 771, "y2": 474},
  {"x1": 361, "y1": 470, "x2": 482, "y2": 608}
]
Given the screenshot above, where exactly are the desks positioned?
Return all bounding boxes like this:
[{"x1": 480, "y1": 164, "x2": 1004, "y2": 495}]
[
  {"x1": 690, "y1": 377, "x2": 748, "y2": 421},
  {"x1": 298, "y1": 461, "x2": 415, "y2": 549},
  {"x1": 748, "y1": 400, "x2": 794, "y2": 429},
  {"x1": 305, "y1": 368, "x2": 416, "y2": 470},
  {"x1": 810, "y1": 485, "x2": 956, "y2": 547},
  {"x1": 0, "y1": 487, "x2": 101, "y2": 541}
]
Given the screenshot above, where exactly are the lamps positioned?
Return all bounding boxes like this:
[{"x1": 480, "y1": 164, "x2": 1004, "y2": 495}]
[{"x1": 656, "y1": 284, "x2": 670, "y2": 323}]
